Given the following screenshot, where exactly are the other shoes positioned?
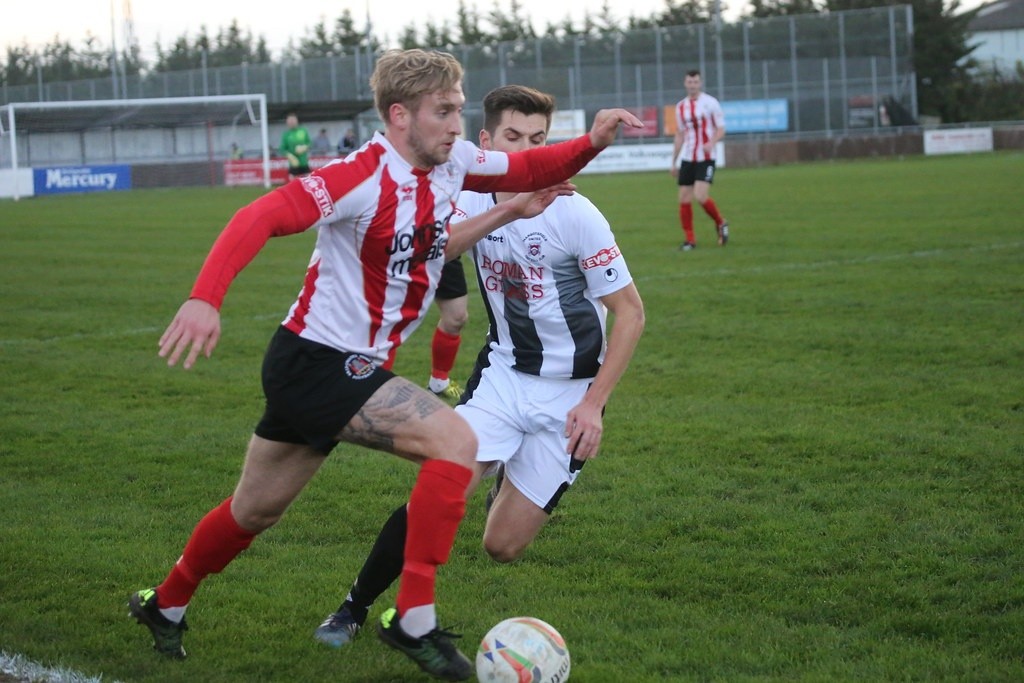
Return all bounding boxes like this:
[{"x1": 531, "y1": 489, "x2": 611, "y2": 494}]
[{"x1": 425, "y1": 379, "x2": 464, "y2": 401}]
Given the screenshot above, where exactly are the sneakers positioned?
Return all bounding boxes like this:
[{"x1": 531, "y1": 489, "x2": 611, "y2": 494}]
[
  {"x1": 125, "y1": 587, "x2": 190, "y2": 661},
  {"x1": 309, "y1": 609, "x2": 365, "y2": 651},
  {"x1": 377, "y1": 607, "x2": 474, "y2": 682},
  {"x1": 716, "y1": 219, "x2": 730, "y2": 245},
  {"x1": 680, "y1": 241, "x2": 696, "y2": 252}
]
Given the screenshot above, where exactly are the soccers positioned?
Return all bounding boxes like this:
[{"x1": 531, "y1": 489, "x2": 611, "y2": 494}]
[{"x1": 475, "y1": 616, "x2": 571, "y2": 683}]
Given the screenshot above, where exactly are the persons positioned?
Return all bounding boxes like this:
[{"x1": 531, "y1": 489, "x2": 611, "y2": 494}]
[
  {"x1": 311, "y1": 129, "x2": 331, "y2": 156},
  {"x1": 314, "y1": 84, "x2": 644, "y2": 648},
  {"x1": 338, "y1": 129, "x2": 357, "y2": 154},
  {"x1": 230, "y1": 142, "x2": 242, "y2": 160},
  {"x1": 128, "y1": 48, "x2": 644, "y2": 683},
  {"x1": 259, "y1": 144, "x2": 277, "y2": 158},
  {"x1": 279, "y1": 112, "x2": 311, "y2": 183},
  {"x1": 432, "y1": 254, "x2": 470, "y2": 401},
  {"x1": 672, "y1": 70, "x2": 729, "y2": 251}
]
